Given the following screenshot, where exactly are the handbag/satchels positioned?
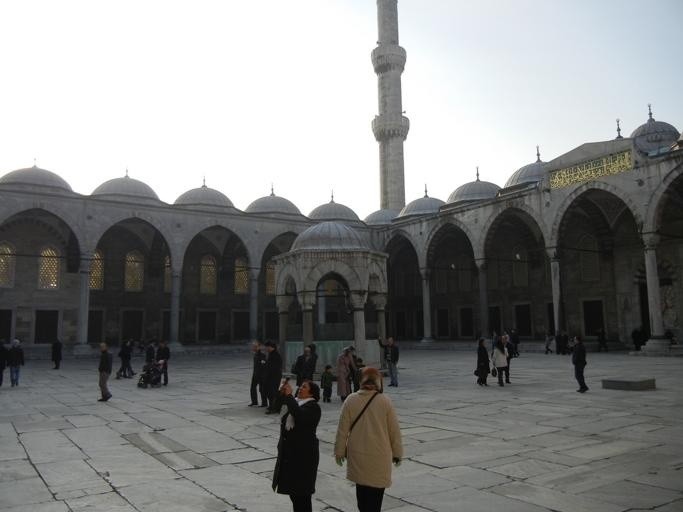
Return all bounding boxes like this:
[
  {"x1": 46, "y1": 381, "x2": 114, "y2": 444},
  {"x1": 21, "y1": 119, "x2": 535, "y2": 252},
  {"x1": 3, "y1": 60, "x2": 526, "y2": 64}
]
[
  {"x1": 474, "y1": 370, "x2": 480, "y2": 376},
  {"x1": 491, "y1": 365, "x2": 497, "y2": 377}
]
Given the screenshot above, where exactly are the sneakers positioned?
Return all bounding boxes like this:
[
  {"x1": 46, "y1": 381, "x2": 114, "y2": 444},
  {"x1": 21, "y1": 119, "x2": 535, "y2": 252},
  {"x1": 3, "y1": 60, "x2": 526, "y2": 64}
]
[
  {"x1": 248, "y1": 403, "x2": 259, "y2": 407},
  {"x1": 97, "y1": 394, "x2": 113, "y2": 402},
  {"x1": 576, "y1": 382, "x2": 589, "y2": 394},
  {"x1": 258, "y1": 405, "x2": 269, "y2": 408},
  {"x1": 387, "y1": 384, "x2": 399, "y2": 387}
]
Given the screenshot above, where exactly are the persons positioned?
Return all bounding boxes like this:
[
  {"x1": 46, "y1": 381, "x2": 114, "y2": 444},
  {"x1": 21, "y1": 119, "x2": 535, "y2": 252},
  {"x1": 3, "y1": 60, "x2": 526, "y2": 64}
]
[
  {"x1": 571, "y1": 331, "x2": 590, "y2": 395},
  {"x1": 247, "y1": 337, "x2": 366, "y2": 416},
  {"x1": 51, "y1": 337, "x2": 62, "y2": 370},
  {"x1": 492, "y1": 323, "x2": 675, "y2": 369},
  {"x1": 490, "y1": 337, "x2": 509, "y2": 388},
  {"x1": 271, "y1": 381, "x2": 322, "y2": 511},
  {"x1": 96, "y1": 342, "x2": 113, "y2": 403},
  {"x1": 377, "y1": 333, "x2": 400, "y2": 390},
  {"x1": 334, "y1": 366, "x2": 405, "y2": 511},
  {"x1": 6, "y1": 338, "x2": 24, "y2": 388},
  {"x1": 115, "y1": 335, "x2": 170, "y2": 388},
  {"x1": 471, "y1": 335, "x2": 490, "y2": 387},
  {"x1": 0, "y1": 338, "x2": 9, "y2": 385}
]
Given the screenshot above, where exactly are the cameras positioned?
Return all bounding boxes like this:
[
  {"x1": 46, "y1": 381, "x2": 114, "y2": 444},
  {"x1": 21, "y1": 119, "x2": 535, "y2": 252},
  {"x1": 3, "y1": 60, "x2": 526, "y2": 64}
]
[{"x1": 280, "y1": 376, "x2": 290, "y2": 391}]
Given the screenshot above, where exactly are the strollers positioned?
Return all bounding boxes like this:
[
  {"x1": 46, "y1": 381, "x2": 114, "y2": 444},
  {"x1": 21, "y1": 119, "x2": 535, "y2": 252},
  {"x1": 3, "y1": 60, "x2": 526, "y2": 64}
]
[{"x1": 136, "y1": 360, "x2": 163, "y2": 389}]
